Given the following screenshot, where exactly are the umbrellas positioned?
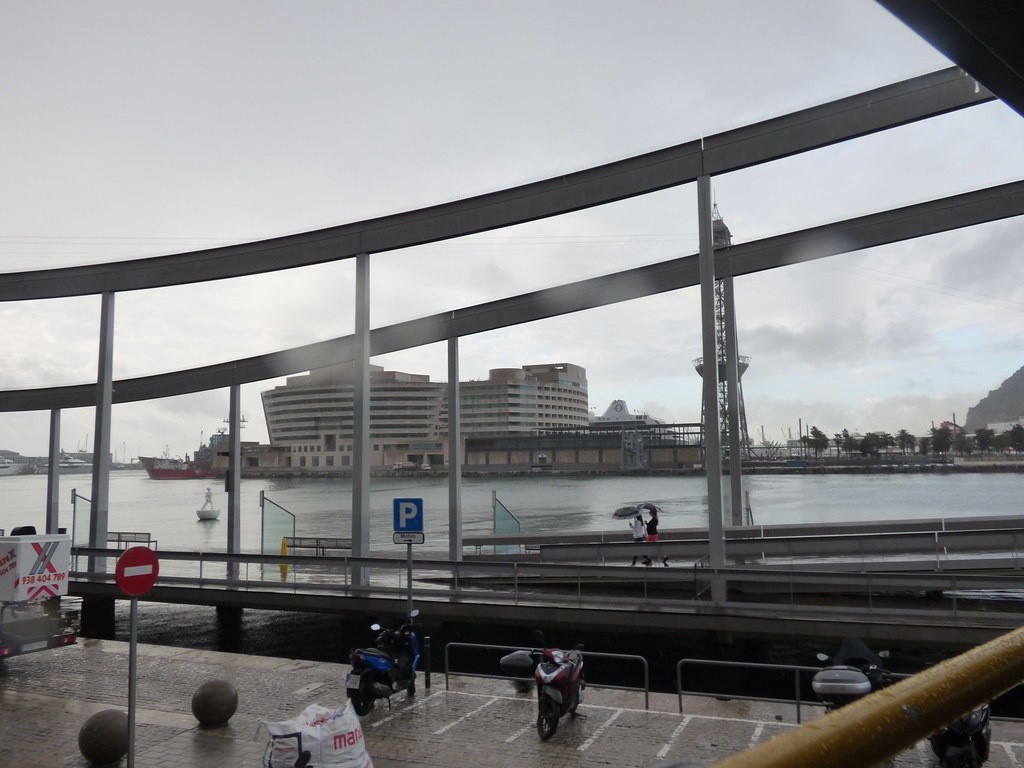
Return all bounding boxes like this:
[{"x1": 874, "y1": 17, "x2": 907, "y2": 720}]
[
  {"x1": 612, "y1": 506, "x2": 643, "y2": 519},
  {"x1": 637, "y1": 502, "x2": 663, "y2": 514}
]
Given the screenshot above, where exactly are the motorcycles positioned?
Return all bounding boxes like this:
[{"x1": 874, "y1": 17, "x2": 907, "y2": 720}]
[{"x1": 345, "y1": 609, "x2": 421, "y2": 718}]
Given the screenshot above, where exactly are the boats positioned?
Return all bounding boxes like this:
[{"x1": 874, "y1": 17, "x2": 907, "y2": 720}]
[
  {"x1": 137, "y1": 453, "x2": 220, "y2": 481},
  {"x1": 0, "y1": 455, "x2": 30, "y2": 476},
  {"x1": 36, "y1": 448, "x2": 94, "y2": 475}
]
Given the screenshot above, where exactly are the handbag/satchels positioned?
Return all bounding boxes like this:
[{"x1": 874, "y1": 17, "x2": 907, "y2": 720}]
[{"x1": 254, "y1": 697, "x2": 373, "y2": 768}]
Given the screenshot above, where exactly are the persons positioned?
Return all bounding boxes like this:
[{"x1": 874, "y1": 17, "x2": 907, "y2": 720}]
[
  {"x1": 642, "y1": 509, "x2": 670, "y2": 567},
  {"x1": 627, "y1": 514, "x2": 651, "y2": 566}
]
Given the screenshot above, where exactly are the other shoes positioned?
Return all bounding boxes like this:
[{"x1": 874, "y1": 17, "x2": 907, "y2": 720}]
[
  {"x1": 663, "y1": 556, "x2": 668, "y2": 563},
  {"x1": 643, "y1": 560, "x2": 651, "y2": 564}
]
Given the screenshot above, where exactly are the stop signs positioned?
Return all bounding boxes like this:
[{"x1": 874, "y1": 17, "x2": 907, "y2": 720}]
[{"x1": 114, "y1": 545, "x2": 160, "y2": 597}]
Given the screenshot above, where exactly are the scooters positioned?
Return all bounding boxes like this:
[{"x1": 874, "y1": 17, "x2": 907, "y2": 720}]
[
  {"x1": 811, "y1": 650, "x2": 892, "y2": 713},
  {"x1": 529, "y1": 629, "x2": 586, "y2": 741},
  {"x1": 922, "y1": 646, "x2": 992, "y2": 768}
]
